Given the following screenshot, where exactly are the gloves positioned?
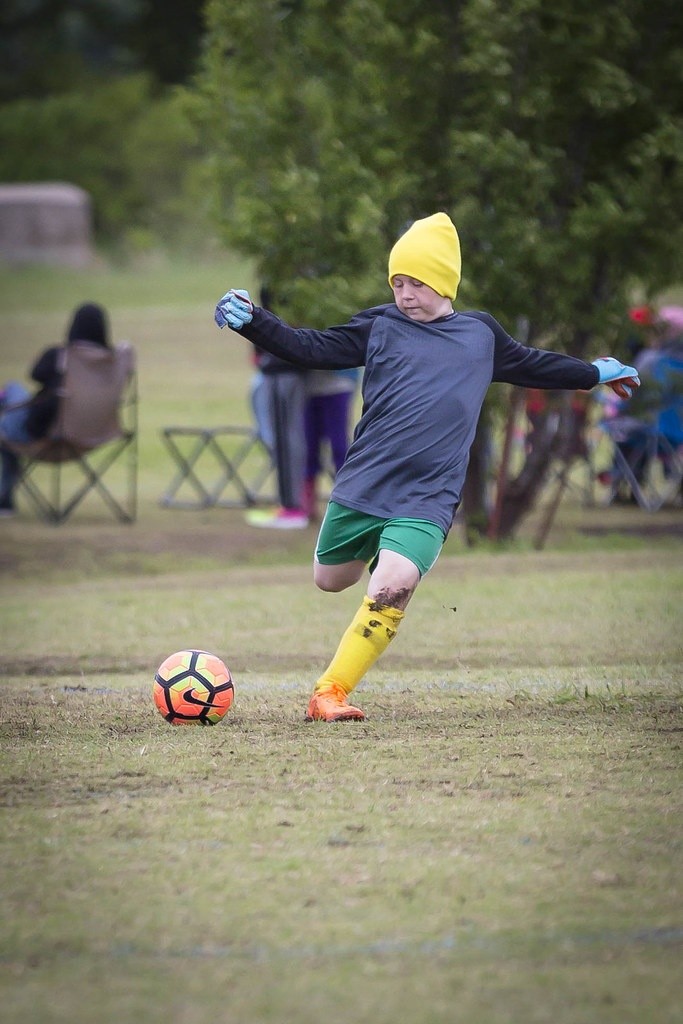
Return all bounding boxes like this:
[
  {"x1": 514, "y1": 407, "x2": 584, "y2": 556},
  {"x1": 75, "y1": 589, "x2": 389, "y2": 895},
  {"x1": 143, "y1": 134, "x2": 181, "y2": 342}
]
[
  {"x1": 214, "y1": 288, "x2": 254, "y2": 332},
  {"x1": 590, "y1": 356, "x2": 641, "y2": 401}
]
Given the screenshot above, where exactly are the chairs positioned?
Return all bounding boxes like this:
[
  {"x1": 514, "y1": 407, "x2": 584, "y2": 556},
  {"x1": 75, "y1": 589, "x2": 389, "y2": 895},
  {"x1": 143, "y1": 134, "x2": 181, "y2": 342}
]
[
  {"x1": 0, "y1": 344, "x2": 139, "y2": 525},
  {"x1": 159, "y1": 384, "x2": 275, "y2": 508}
]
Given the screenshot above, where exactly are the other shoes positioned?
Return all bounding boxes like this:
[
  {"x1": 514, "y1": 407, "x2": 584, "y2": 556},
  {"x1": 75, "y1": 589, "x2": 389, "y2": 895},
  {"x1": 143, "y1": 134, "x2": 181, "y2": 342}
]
[
  {"x1": 629, "y1": 491, "x2": 640, "y2": 506},
  {"x1": 607, "y1": 493, "x2": 629, "y2": 510},
  {"x1": 0, "y1": 506, "x2": 19, "y2": 520}
]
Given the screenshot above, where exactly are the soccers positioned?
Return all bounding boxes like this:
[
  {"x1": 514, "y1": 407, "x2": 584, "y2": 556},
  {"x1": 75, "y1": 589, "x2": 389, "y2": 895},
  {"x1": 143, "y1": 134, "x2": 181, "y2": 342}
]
[{"x1": 153, "y1": 649, "x2": 235, "y2": 725}]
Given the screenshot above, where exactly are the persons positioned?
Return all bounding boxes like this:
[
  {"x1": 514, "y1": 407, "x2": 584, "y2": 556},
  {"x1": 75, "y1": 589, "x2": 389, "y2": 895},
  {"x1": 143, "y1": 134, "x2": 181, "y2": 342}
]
[
  {"x1": 1, "y1": 302, "x2": 112, "y2": 516},
  {"x1": 244, "y1": 278, "x2": 683, "y2": 529},
  {"x1": 213, "y1": 212, "x2": 642, "y2": 724}
]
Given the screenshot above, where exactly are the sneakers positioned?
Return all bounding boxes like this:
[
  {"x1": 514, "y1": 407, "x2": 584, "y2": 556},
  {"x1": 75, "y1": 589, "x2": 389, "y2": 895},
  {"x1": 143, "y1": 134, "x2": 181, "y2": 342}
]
[
  {"x1": 241, "y1": 507, "x2": 311, "y2": 533},
  {"x1": 302, "y1": 682, "x2": 365, "y2": 724}
]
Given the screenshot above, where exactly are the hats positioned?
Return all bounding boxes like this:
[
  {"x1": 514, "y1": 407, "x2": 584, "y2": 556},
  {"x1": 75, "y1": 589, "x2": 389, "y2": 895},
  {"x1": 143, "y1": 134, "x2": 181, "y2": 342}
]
[
  {"x1": 387, "y1": 210, "x2": 462, "y2": 302},
  {"x1": 657, "y1": 306, "x2": 683, "y2": 332},
  {"x1": 627, "y1": 307, "x2": 656, "y2": 326}
]
